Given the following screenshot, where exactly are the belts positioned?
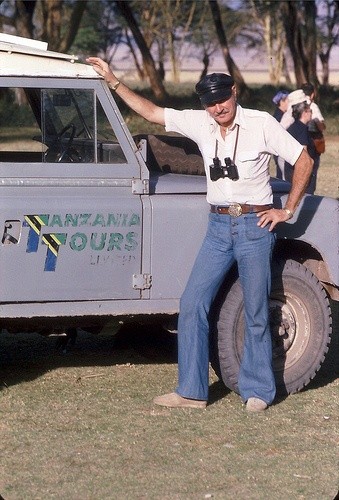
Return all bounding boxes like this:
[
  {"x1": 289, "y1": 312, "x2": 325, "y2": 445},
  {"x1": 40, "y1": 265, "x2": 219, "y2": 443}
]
[{"x1": 210, "y1": 203, "x2": 274, "y2": 217}]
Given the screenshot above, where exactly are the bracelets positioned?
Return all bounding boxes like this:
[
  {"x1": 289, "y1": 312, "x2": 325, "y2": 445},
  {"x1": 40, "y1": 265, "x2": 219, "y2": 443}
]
[
  {"x1": 111, "y1": 82, "x2": 120, "y2": 90},
  {"x1": 283, "y1": 208, "x2": 293, "y2": 218}
]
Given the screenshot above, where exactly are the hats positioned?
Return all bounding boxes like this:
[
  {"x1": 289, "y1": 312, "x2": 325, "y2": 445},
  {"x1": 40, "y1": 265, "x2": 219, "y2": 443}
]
[
  {"x1": 286, "y1": 89, "x2": 311, "y2": 112},
  {"x1": 272, "y1": 92, "x2": 286, "y2": 104},
  {"x1": 195, "y1": 73, "x2": 235, "y2": 105}
]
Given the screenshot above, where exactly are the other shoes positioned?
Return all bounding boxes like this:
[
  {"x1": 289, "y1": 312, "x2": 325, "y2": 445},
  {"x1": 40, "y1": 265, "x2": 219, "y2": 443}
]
[
  {"x1": 154, "y1": 392, "x2": 207, "y2": 407},
  {"x1": 247, "y1": 397, "x2": 267, "y2": 411}
]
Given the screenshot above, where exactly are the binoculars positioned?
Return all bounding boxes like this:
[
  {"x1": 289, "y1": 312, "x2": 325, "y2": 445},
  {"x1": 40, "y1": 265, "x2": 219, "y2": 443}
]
[{"x1": 210, "y1": 157, "x2": 239, "y2": 181}]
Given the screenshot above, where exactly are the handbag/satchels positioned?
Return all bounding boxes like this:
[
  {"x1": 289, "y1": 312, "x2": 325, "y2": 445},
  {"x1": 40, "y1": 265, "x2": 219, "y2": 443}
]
[{"x1": 310, "y1": 137, "x2": 325, "y2": 153}]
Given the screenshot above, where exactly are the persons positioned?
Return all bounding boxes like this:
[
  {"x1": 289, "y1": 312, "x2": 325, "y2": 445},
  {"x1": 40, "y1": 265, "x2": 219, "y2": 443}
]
[
  {"x1": 301, "y1": 83, "x2": 326, "y2": 140},
  {"x1": 278, "y1": 89, "x2": 310, "y2": 181},
  {"x1": 86, "y1": 56, "x2": 314, "y2": 410},
  {"x1": 283, "y1": 101, "x2": 319, "y2": 194},
  {"x1": 273, "y1": 91, "x2": 289, "y2": 179}
]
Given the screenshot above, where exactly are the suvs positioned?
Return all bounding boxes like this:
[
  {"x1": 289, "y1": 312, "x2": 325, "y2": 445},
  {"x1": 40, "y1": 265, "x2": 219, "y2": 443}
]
[{"x1": 0, "y1": 30, "x2": 339, "y2": 400}]
